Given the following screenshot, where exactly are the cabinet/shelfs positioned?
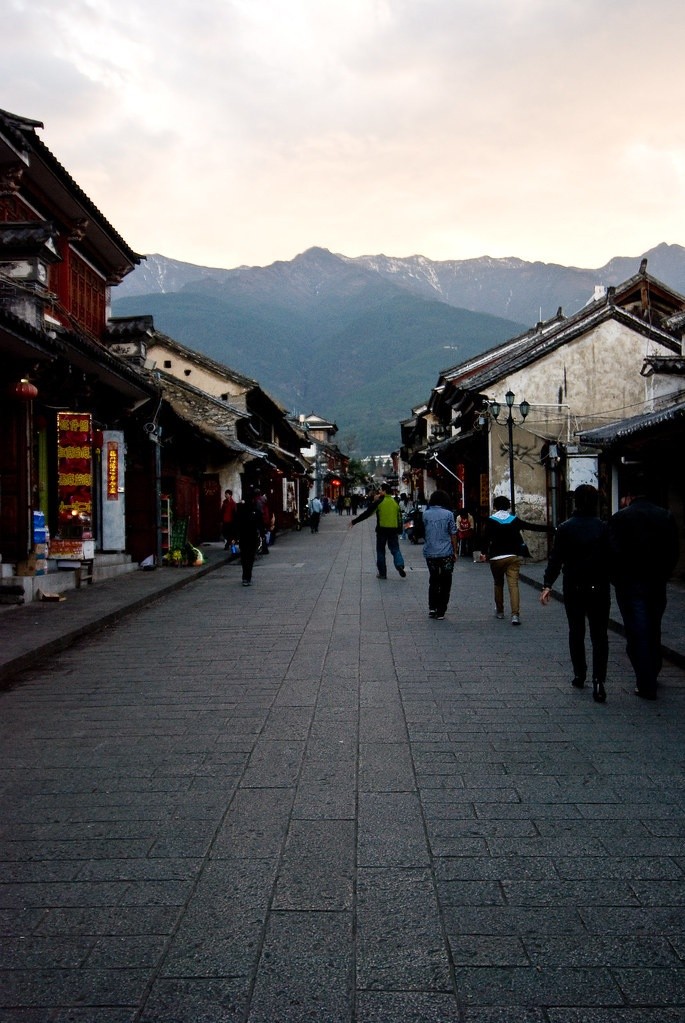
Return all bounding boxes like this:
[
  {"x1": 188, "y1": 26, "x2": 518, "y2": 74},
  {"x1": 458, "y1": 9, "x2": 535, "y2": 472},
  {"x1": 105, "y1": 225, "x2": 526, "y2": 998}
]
[{"x1": 161, "y1": 498, "x2": 170, "y2": 550}]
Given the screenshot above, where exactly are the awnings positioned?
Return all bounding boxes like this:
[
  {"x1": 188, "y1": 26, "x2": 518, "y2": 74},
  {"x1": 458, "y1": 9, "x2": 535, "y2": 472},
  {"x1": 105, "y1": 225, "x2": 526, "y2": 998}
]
[
  {"x1": 577, "y1": 401, "x2": 685, "y2": 461},
  {"x1": 409, "y1": 430, "x2": 486, "y2": 483}
]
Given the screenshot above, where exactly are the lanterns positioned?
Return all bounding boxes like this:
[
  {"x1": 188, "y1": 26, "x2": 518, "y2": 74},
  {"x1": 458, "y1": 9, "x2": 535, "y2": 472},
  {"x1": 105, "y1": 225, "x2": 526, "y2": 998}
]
[{"x1": 10, "y1": 382, "x2": 38, "y2": 402}]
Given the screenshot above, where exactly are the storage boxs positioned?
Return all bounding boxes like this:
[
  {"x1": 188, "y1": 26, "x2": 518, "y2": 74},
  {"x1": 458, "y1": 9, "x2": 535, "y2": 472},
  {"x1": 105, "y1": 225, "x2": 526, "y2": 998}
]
[{"x1": 50, "y1": 539, "x2": 94, "y2": 559}]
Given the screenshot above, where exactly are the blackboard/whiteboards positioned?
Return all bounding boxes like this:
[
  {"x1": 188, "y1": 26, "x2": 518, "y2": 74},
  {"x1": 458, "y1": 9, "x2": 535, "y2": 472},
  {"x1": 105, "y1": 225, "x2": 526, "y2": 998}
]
[{"x1": 170, "y1": 515, "x2": 191, "y2": 551}]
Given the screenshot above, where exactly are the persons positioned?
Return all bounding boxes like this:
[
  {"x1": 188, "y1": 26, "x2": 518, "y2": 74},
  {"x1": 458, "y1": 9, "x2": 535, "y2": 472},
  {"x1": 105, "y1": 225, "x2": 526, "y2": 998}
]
[
  {"x1": 309, "y1": 494, "x2": 331, "y2": 534},
  {"x1": 456, "y1": 509, "x2": 477, "y2": 555},
  {"x1": 538, "y1": 484, "x2": 681, "y2": 700},
  {"x1": 334, "y1": 484, "x2": 413, "y2": 579},
  {"x1": 220, "y1": 487, "x2": 276, "y2": 587},
  {"x1": 481, "y1": 496, "x2": 551, "y2": 625},
  {"x1": 422, "y1": 491, "x2": 458, "y2": 620}
]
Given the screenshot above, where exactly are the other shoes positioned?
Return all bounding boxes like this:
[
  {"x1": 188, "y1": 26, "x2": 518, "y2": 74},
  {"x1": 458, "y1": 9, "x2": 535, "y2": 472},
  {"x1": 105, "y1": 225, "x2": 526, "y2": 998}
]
[
  {"x1": 635, "y1": 687, "x2": 658, "y2": 701},
  {"x1": 376, "y1": 573, "x2": 387, "y2": 579},
  {"x1": 495, "y1": 613, "x2": 504, "y2": 618},
  {"x1": 399, "y1": 567, "x2": 406, "y2": 577},
  {"x1": 593, "y1": 678, "x2": 606, "y2": 704},
  {"x1": 429, "y1": 610, "x2": 437, "y2": 618},
  {"x1": 572, "y1": 677, "x2": 584, "y2": 688},
  {"x1": 242, "y1": 580, "x2": 252, "y2": 585},
  {"x1": 511, "y1": 615, "x2": 519, "y2": 624},
  {"x1": 437, "y1": 614, "x2": 445, "y2": 620}
]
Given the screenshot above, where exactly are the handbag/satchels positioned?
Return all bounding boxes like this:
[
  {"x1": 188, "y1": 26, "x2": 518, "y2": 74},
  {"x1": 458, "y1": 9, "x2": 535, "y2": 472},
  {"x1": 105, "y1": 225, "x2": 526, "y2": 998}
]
[{"x1": 398, "y1": 522, "x2": 403, "y2": 534}]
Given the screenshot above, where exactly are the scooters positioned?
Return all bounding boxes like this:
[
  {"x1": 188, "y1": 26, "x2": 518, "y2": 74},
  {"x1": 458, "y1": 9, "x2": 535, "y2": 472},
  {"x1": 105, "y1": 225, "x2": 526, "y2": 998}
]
[{"x1": 401, "y1": 501, "x2": 425, "y2": 545}]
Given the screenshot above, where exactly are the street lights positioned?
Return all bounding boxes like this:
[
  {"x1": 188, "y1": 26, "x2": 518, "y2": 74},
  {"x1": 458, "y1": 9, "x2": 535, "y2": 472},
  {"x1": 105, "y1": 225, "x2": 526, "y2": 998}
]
[{"x1": 489, "y1": 390, "x2": 530, "y2": 517}]
